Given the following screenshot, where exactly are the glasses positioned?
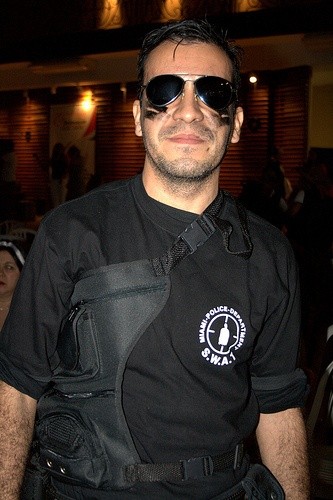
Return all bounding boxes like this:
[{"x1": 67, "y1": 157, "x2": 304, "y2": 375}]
[{"x1": 139, "y1": 74, "x2": 237, "y2": 111}]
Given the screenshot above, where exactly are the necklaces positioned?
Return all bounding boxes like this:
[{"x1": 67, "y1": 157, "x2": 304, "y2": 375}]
[{"x1": 0, "y1": 304, "x2": 9, "y2": 312}]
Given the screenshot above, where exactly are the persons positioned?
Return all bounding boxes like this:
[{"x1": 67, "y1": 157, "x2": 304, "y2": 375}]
[
  {"x1": 0, "y1": 140, "x2": 333, "y2": 454},
  {"x1": 0, "y1": 22, "x2": 312, "y2": 500}
]
